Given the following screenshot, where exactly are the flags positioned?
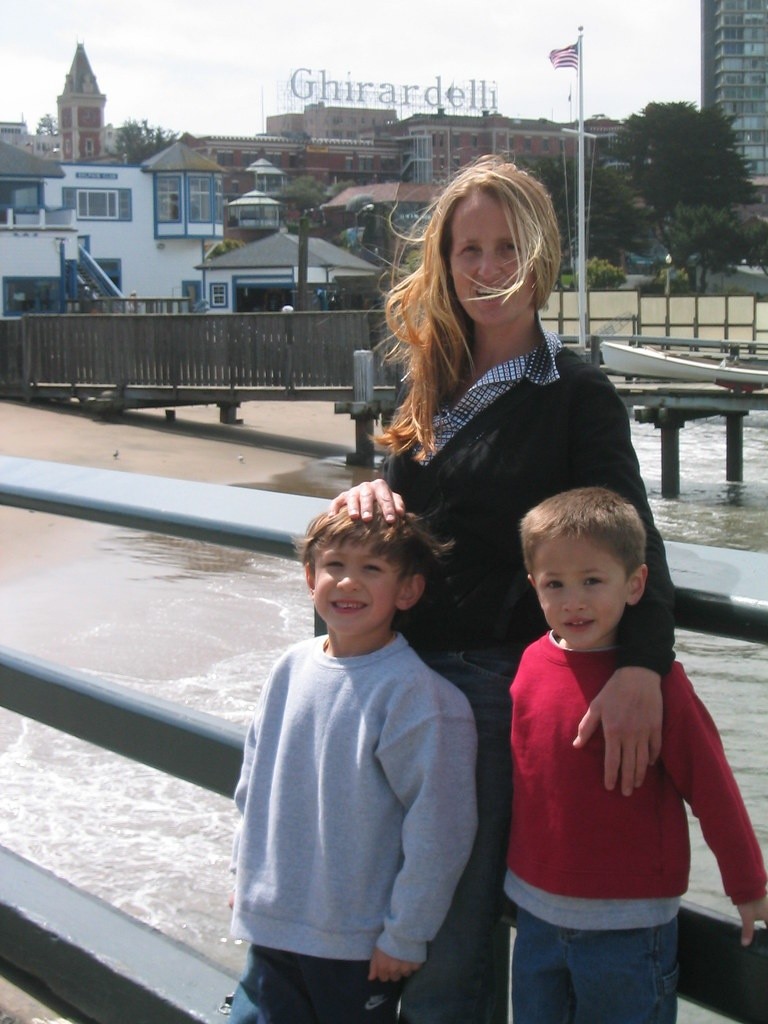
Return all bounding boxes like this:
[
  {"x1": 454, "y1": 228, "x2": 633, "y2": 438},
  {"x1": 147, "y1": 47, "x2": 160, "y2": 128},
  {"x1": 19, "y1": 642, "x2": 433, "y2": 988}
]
[{"x1": 549, "y1": 43, "x2": 578, "y2": 71}]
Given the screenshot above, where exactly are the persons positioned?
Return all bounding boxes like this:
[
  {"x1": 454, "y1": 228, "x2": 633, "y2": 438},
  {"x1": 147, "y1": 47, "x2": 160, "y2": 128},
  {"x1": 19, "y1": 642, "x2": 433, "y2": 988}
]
[
  {"x1": 501, "y1": 486, "x2": 768, "y2": 1024},
  {"x1": 328, "y1": 156, "x2": 675, "y2": 1024},
  {"x1": 225, "y1": 500, "x2": 479, "y2": 1024}
]
